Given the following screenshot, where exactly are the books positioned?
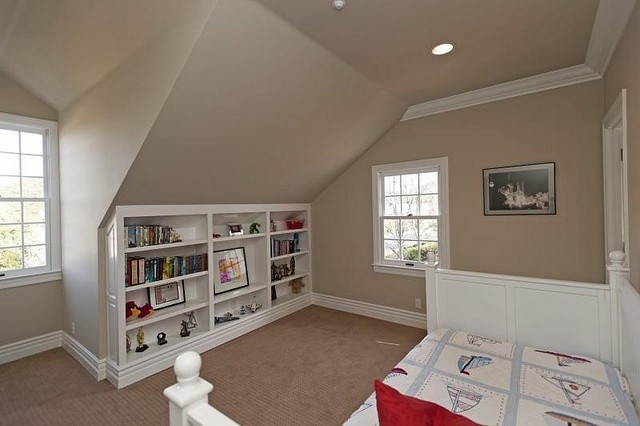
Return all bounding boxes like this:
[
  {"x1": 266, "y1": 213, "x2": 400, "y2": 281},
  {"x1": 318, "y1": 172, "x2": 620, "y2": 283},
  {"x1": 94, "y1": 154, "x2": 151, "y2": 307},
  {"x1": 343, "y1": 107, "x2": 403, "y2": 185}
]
[
  {"x1": 126, "y1": 252, "x2": 208, "y2": 289},
  {"x1": 270, "y1": 233, "x2": 299, "y2": 258},
  {"x1": 125, "y1": 225, "x2": 182, "y2": 249}
]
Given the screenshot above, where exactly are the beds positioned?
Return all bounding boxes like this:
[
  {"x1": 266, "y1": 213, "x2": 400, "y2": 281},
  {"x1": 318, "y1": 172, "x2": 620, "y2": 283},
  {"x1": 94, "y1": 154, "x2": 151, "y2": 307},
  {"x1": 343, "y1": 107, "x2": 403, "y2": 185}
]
[{"x1": 164, "y1": 248, "x2": 640, "y2": 426}]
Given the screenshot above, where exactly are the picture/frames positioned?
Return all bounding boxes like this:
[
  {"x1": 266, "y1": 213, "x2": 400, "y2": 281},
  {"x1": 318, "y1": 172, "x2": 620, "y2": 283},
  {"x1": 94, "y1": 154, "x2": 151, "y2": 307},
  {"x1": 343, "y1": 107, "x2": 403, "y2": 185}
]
[
  {"x1": 481, "y1": 160, "x2": 559, "y2": 217},
  {"x1": 209, "y1": 245, "x2": 251, "y2": 295},
  {"x1": 145, "y1": 281, "x2": 186, "y2": 310}
]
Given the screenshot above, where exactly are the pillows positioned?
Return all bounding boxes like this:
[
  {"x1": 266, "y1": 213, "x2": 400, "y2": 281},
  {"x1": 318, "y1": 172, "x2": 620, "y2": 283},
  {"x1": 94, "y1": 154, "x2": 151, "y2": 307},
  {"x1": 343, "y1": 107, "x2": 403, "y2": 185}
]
[{"x1": 373, "y1": 378, "x2": 487, "y2": 426}]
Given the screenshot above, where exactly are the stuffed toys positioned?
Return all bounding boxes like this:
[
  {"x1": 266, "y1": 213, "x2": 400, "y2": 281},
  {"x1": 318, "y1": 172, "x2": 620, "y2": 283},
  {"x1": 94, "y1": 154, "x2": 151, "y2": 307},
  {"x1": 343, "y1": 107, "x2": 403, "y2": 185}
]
[
  {"x1": 126, "y1": 301, "x2": 141, "y2": 322},
  {"x1": 137, "y1": 303, "x2": 155, "y2": 319}
]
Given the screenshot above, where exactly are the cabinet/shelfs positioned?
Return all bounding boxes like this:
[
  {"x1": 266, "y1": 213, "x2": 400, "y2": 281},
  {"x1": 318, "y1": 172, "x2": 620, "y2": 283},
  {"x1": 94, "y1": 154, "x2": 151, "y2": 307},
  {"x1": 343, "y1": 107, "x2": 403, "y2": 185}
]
[
  {"x1": 269, "y1": 203, "x2": 311, "y2": 325},
  {"x1": 101, "y1": 205, "x2": 210, "y2": 391},
  {"x1": 211, "y1": 204, "x2": 271, "y2": 351}
]
